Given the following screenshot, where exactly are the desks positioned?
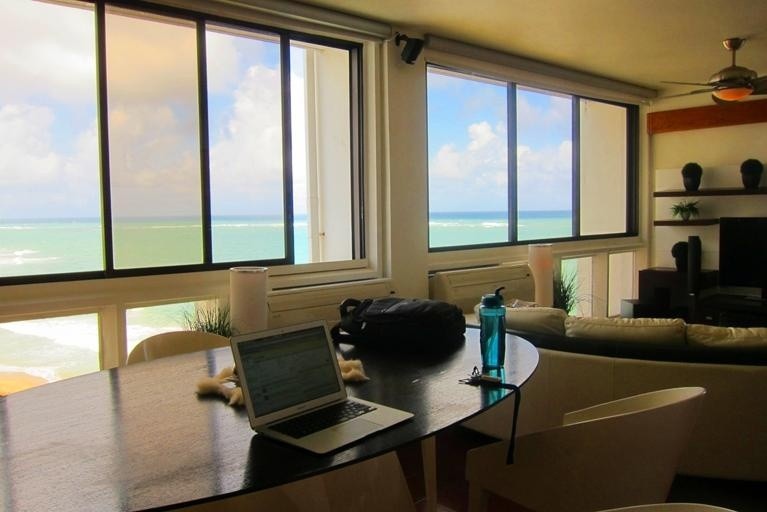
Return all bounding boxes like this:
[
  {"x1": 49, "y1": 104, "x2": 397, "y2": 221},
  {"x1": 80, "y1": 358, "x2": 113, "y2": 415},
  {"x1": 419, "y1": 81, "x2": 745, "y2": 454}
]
[{"x1": 0, "y1": 313, "x2": 544, "y2": 509}]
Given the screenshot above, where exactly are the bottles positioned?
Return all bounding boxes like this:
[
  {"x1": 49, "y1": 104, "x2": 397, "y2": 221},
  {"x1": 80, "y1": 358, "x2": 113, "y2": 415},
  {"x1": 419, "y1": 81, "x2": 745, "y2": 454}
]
[{"x1": 478, "y1": 284, "x2": 508, "y2": 370}]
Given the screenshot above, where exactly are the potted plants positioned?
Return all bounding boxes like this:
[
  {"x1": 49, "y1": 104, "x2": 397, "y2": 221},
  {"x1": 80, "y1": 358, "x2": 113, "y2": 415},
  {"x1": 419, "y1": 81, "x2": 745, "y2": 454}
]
[
  {"x1": 670, "y1": 241, "x2": 689, "y2": 271},
  {"x1": 680, "y1": 162, "x2": 703, "y2": 190},
  {"x1": 669, "y1": 200, "x2": 700, "y2": 220},
  {"x1": 739, "y1": 159, "x2": 764, "y2": 187}
]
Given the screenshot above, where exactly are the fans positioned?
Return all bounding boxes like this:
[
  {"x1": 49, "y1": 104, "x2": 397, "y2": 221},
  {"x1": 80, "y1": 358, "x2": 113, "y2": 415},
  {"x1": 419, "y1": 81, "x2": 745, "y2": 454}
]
[{"x1": 653, "y1": 37, "x2": 767, "y2": 101}]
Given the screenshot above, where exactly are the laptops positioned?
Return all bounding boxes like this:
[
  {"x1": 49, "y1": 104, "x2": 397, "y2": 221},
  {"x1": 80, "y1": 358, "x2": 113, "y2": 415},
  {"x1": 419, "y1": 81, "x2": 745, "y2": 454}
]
[{"x1": 230, "y1": 318, "x2": 415, "y2": 456}]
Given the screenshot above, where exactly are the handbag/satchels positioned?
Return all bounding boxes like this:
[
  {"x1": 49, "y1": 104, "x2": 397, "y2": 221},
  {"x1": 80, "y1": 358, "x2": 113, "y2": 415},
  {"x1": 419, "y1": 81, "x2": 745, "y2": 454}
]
[{"x1": 330, "y1": 298, "x2": 466, "y2": 354}]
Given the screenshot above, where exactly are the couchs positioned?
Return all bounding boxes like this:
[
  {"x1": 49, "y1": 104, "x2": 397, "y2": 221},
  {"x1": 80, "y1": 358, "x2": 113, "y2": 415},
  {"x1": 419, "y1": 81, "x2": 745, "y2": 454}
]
[{"x1": 435, "y1": 263, "x2": 767, "y2": 480}]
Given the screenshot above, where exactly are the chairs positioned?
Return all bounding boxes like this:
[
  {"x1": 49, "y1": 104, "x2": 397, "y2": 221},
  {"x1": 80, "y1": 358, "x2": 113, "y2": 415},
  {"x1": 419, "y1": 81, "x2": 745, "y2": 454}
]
[
  {"x1": 466, "y1": 384, "x2": 706, "y2": 501},
  {"x1": 126, "y1": 331, "x2": 237, "y2": 364}
]
